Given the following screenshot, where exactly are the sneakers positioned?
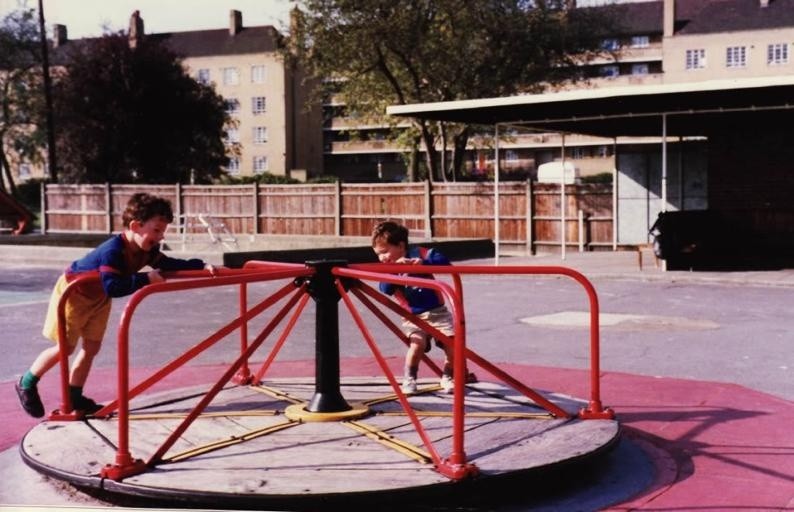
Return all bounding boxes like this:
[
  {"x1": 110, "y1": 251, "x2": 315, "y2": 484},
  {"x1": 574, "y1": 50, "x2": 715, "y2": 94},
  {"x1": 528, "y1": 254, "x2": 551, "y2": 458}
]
[
  {"x1": 440, "y1": 375, "x2": 455, "y2": 394},
  {"x1": 14, "y1": 375, "x2": 45, "y2": 419},
  {"x1": 68, "y1": 396, "x2": 104, "y2": 413},
  {"x1": 401, "y1": 376, "x2": 418, "y2": 394}
]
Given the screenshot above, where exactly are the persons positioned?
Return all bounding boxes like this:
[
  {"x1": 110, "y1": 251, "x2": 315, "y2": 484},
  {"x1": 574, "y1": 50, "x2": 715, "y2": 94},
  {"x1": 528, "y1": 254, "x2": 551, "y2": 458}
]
[
  {"x1": 10, "y1": 191, "x2": 220, "y2": 422},
  {"x1": 366, "y1": 220, "x2": 466, "y2": 396}
]
